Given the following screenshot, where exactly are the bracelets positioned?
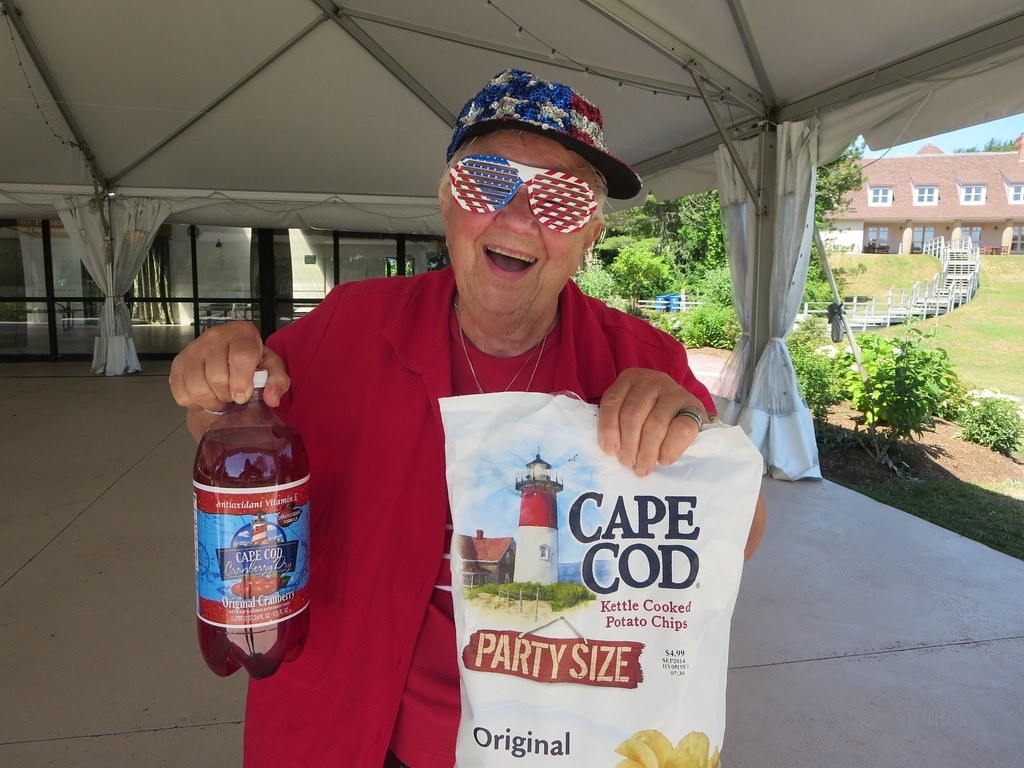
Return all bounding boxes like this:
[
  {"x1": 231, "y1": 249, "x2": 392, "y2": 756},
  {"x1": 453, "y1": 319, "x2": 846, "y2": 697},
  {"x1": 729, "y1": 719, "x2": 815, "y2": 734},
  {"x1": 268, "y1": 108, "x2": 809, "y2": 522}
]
[{"x1": 202, "y1": 407, "x2": 226, "y2": 416}]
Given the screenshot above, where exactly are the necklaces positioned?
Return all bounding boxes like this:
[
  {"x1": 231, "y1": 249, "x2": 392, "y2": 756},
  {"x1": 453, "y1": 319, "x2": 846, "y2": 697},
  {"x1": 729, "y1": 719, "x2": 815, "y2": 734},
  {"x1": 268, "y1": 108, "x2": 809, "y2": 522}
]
[{"x1": 456, "y1": 303, "x2": 548, "y2": 395}]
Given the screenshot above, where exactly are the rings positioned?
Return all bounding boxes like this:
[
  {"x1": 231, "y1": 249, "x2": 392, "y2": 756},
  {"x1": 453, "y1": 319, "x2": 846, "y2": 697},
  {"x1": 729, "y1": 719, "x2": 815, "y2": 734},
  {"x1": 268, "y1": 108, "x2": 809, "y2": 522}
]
[{"x1": 676, "y1": 408, "x2": 703, "y2": 431}]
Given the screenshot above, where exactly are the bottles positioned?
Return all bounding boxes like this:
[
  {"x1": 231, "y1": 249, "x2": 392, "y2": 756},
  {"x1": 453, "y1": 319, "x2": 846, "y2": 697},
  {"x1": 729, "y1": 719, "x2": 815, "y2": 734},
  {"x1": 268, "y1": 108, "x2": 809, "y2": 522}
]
[{"x1": 191, "y1": 367, "x2": 311, "y2": 679}]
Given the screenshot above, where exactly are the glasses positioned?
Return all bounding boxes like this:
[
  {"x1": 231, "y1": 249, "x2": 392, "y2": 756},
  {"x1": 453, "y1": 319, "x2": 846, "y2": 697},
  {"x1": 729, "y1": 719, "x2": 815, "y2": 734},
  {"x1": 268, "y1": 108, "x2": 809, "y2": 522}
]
[{"x1": 449, "y1": 154, "x2": 598, "y2": 234}]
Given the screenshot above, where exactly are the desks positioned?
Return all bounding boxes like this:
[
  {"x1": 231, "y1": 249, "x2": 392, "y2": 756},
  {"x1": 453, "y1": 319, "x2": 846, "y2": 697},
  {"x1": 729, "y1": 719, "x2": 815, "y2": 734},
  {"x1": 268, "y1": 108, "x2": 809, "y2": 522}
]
[
  {"x1": 193, "y1": 305, "x2": 252, "y2": 327},
  {"x1": 20, "y1": 309, "x2": 76, "y2": 330}
]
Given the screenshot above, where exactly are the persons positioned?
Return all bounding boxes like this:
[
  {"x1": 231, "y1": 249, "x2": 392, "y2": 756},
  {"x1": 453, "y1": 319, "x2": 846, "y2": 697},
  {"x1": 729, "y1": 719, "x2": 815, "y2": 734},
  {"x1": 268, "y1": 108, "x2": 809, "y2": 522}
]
[{"x1": 167, "y1": 69, "x2": 770, "y2": 768}]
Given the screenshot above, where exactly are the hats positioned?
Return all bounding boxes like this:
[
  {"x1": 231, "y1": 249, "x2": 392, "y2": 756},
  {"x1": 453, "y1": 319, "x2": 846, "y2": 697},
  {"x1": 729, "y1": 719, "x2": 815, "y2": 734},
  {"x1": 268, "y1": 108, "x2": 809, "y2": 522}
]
[{"x1": 446, "y1": 66, "x2": 645, "y2": 199}]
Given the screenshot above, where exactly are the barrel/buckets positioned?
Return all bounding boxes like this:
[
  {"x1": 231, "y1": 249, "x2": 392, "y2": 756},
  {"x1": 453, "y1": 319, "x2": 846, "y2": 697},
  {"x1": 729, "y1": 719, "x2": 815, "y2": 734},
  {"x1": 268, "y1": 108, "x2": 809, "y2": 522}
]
[{"x1": 656, "y1": 295, "x2": 681, "y2": 313}]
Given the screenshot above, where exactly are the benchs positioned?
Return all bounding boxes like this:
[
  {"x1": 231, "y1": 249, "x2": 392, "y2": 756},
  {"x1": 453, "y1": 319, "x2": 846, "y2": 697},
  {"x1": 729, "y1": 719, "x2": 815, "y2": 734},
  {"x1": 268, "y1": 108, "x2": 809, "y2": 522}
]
[{"x1": 190, "y1": 316, "x2": 252, "y2": 331}]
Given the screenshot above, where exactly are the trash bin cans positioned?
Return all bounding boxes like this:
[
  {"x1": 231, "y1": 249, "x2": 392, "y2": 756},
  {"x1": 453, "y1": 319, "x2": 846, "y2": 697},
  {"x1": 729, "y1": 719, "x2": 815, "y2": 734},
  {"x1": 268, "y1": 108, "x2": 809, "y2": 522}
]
[{"x1": 655, "y1": 294, "x2": 681, "y2": 312}]
